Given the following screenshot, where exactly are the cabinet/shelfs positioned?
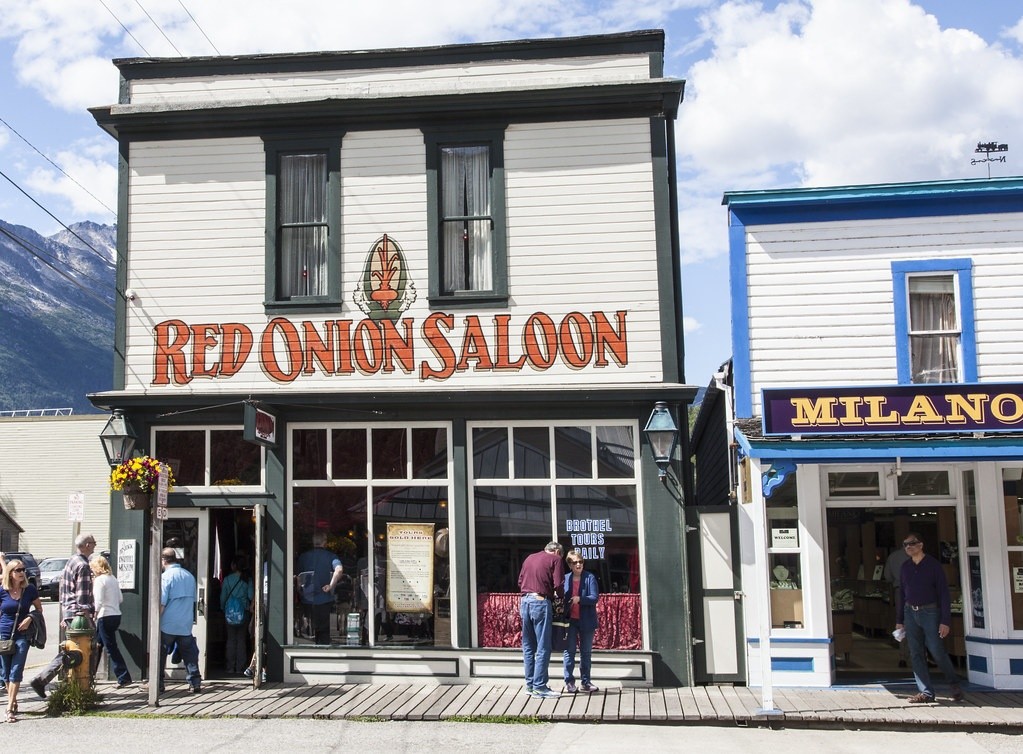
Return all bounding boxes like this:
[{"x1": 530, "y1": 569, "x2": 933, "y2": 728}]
[{"x1": 831, "y1": 577, "x2": 987, "y2": 670}]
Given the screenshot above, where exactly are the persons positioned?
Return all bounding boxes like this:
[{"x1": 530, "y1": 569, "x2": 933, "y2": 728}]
[
  {"x1": 30, "y1": 532, "x2": 97, "y2": 699},
  {"x1": 293, "y1": 574, "x2": 316, "y2": 640},
  {"x1": 875, "y1": 531, "x2": 969, "y2": 705},
  {"x1": 334, "y1": 556, "x2": 394, "y2": 641},
  {"x1": 518, "y1": 541, "x2": 565, "y2": 695},
  {"x1": 220, "y1": 557, "x2": 265, "y2": 680},
  {"x1": 89, "y1": 556, "x2": 132, "y2": 690},
  {"x1": 553, "y1": 551, "x2": 599, "y2": 693},
  {"x1": 157, "y1": 547, "x2": 201, "y2": 694},
  {"x1": 165, "y1": 537, "x2": 196, "y2": 586},
  {"x1": 293, "y1": 532, "x2": 343, "y2": 645},
  {"x1": 0, "y1": 551, "x2": 43, "y2": 723}
]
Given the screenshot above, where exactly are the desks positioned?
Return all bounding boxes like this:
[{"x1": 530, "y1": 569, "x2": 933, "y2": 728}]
[{"x1": 771, "y1": 589, "x2": 802, "y2": 626}]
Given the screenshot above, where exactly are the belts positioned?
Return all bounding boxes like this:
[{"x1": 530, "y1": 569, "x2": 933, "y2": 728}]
[
  {"x1": 527, "y1": 593, "x2": 542, "y2": 596},
  {"x1": 905, "y1": 603, "x2": 936, "y2": 610}
]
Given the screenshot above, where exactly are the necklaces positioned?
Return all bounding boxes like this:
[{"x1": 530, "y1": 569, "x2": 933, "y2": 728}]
[{"x1": 13, "y1": 591, "x2": 17, "y2": 593}]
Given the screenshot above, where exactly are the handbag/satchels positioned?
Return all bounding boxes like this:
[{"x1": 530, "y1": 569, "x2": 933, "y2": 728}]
[
  {"x1": 550, "y1": 599, "x2": 574, "y2": 651},
  {"x1": 0, "y1": 639, "x2": 15, "y2": 655}
]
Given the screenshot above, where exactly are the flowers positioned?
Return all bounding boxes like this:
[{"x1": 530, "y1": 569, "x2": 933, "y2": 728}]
[
  {"x1": 110, "y1": 455, "x2": 175, "y2": 494},
  {"x1": 214, "y1": 478, "x2": 245, "y2": 487}
]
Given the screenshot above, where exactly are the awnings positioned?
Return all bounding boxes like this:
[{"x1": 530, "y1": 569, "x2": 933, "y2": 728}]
[
  {"x1": 87, "y1": 382, "x2": 701, "y2": 410},
  {"x1": 732, "y1": 426, "x2": 1023, "y2": 457}
]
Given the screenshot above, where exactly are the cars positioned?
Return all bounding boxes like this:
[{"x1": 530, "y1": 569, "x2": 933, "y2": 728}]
[
  {"x1": 39, "y1": 558, "x2": 75, "y2": 600},
  {"x1": 0, "y1": 550, "x2": 43, "y2": 600}
]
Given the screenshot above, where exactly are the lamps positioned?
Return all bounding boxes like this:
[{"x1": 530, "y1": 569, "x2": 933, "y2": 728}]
[
  {"x1": 99, "y1": 409, "x2": 138, "y2": 468},
  {"x1": 643, "y1": 402, "x2": 679, "y2": 484}
]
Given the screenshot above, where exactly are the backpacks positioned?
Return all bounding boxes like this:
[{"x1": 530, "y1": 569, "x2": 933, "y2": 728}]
[{"x1": 226, "y1": 597, "x2": 244, "y2": 626}]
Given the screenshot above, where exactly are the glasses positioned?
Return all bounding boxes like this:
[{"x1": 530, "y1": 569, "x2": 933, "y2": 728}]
[
  {"x1": 570, "y1": 560, "x2": 584, "y2": 565},
  {"x1": 902, "y1": 541, "x2": 921, "y2": 547},
  {"x1": 12, "y1": 568, "x2": 26, "y2": 572},
  {"x1": 87, "y1": 542, "x2": 96, "y2": 546}
]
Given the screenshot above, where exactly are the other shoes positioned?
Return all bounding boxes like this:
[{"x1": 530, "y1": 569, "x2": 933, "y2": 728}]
[
  {"x1": 567, "y1": 682, "x2": 578, "y2": 693},
  {"x1": 31, "y1": 678, "x2": 46, "y2": 698},
  {"x1": 952, "y1": 695, "x2": 964, "y2": 702},
  {"x1": 526, "y1": 689, "x2": 561, "y2": 699},
  {"x1": 908, "y1": 693, "x2": 936, "y2": 703},
  {"x1": 581, "y1": 683, "x2": 599, "y2": 692},
  {"x1": 113, "y1": 681, "x2": 132, "y2": 689},
  {"x1": 4, "y1": 703, "x2": 18, "y2": 723},
  {"x1": 262, "y1": 674, "x2": 269, "y2": 682},
  {"x1": 244, "y1": 667, "x2": 253, "y2": 677},
  {"x1": 138, "y1": 682, "x2": 165, "y2": 692},
  {"x1": 186, "y1": 687, "x2": 201, "y2": 693}
]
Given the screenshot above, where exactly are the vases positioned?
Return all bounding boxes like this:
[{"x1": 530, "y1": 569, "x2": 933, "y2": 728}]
[{"x1": 123, "y1": 484, "x2": 149, "y2": 510}]
